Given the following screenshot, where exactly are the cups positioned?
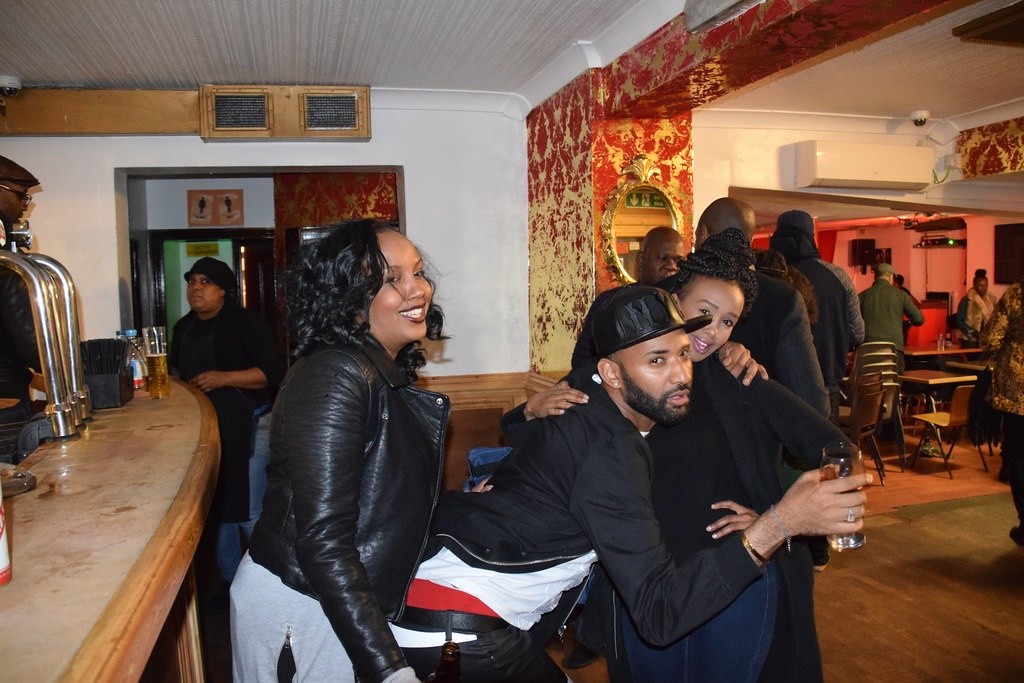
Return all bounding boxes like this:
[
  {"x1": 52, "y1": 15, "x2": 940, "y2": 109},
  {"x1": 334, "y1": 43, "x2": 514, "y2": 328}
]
[
  {"x1": 136, "y1": 337, "x2": 148, "y2": 391},
  {"x1": 820, "y1": 442, "x2": 866, "y2": 550},
  {"x1": 142, "y1": 326, "x2": 171, "y2": 399}
]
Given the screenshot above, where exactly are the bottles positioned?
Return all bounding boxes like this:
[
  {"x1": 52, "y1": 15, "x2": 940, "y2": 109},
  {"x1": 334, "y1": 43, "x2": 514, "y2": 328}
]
[
  {"x1": 116, "y1": 330, "x2": 124, "y2": 340},
  {"x1": 124, "y1": 329, "x2": 144, "y2": 389},
  {"x1": 423, "y1": 641, "x2": 460, "y2": 683},
  {"x1": 937, "y1": 334, "x2": 945, "y2": 351}
]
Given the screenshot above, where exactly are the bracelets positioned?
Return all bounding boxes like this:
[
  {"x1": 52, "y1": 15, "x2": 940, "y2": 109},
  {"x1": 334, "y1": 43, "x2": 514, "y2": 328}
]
[
  {"x1": 739, "y1": 532, "x2": 769, "y2": 567},
  {"x1": 771, "y1": 503, "x2": 793, "y2": 556}
]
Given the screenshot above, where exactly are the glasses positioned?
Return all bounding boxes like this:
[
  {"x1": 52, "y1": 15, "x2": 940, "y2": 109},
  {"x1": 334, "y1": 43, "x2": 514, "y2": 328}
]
[{"x1": 0, "y1": 184, "x2": 32, "y2": 207}]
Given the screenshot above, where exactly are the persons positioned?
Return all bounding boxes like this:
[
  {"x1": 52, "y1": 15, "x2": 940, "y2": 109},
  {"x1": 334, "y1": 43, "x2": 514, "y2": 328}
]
[
  {"x1": 170, "y1": 256, "x2": 283, "y2": 616},
  {"x1": 0, "y1": 153, "x2": 48, "y2": 426},
  {"x1": 500, "y1": 226, "x2": 854, "y2": 683},
  {"x1": 562, "y1": 198, "x2": 1024, "y2": 669},
  {"x1": 229, "y1": 220, "x2": 449, "y2": 683},
  {"x1": 389, "y1": 286, "x2": 874, "y2": 683}
]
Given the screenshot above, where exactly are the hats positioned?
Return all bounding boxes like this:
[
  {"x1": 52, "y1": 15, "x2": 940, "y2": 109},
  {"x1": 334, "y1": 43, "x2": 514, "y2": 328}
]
[
  {"x1": 184, "y1": 257, "x2": 236, "y2": 290},
  {"x1": 0, "y1": 155, "x2": 40, "y2": 187},
  {"x1": 776, "y1": 209, "x2": 815, "y2": 234},
  {"x1": 591, "y1": 284, "x2": 713, "y2": 360},
  {"x1": 874, "y1": 263, "x2": 897, "y2": 278}
]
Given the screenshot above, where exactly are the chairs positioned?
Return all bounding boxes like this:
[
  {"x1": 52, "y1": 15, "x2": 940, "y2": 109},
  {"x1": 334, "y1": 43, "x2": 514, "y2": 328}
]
[
  {"x1": 833, "y1": 341, "x2": 907, "y2": 488},
  {"x1": 909, "y1": 384, "x2": 989, "y2": 476}
]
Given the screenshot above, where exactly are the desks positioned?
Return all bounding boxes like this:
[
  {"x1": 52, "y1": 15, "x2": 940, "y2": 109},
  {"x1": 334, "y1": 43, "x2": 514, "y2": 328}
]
[{"x1": 898, "y1": 346, "x2": 991, "y2": 456}]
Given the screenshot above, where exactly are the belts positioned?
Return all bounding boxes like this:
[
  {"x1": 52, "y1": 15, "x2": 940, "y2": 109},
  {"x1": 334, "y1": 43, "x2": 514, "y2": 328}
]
[{"x1": 390, "y1": 603, "x2": 508, "y2": 637}]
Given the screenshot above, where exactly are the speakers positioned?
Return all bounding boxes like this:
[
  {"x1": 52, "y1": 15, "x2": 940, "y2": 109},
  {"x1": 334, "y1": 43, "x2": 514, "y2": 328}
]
[{"x1": 851, "y1": 239, "x2": 875, "y2": 266}]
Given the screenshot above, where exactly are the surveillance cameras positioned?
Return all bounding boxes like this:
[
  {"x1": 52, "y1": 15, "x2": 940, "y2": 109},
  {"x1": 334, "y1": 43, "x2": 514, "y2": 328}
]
[
  {"x1": 910, "y1": 111, "x2": 929, "y2": 126},
  {"x1": 0, "y1": 76, "x2": 22, "y2": 96}
]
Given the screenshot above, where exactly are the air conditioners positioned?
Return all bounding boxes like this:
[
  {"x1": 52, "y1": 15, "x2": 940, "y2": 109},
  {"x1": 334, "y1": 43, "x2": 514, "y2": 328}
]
[{"x1": 795, "y1": 139, "x2": 936, "y2": 191}]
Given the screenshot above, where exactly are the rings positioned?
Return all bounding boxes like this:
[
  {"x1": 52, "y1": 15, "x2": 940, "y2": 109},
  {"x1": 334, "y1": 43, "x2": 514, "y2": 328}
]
[{"x1": 848, "y1": 507, "x2": 855, "y2": 523}]
